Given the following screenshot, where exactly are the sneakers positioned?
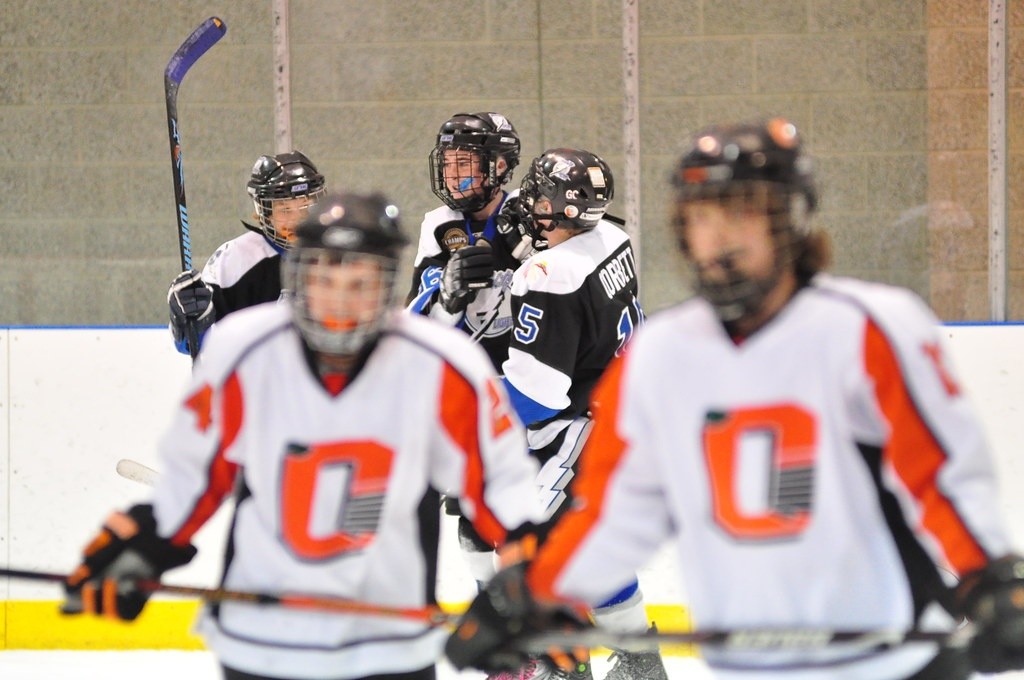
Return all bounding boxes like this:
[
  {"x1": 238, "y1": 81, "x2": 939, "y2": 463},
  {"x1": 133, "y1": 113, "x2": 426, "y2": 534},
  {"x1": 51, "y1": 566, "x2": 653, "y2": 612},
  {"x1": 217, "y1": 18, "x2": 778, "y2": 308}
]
[
  {"x1": 485, "y1": 652, "x2": 594, "y2": 680},
  {"x1": 602, "y1": 621, "x2": 669, "y2": 680}
]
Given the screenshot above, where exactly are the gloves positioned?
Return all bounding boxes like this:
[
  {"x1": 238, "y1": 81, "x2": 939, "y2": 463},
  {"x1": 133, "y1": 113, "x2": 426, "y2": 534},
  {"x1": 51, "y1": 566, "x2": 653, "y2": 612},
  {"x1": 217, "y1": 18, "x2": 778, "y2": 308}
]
[
  {"x1": 167, "y1": 269, "x2": 216, "y2": 342},
  {"x1": 444, "y1": 560, "x2": 598, "y2": 674},
  {"x1": 950, "y1": 554, "x2": 1024, "y2": 674},
  {"x1": 493, "y1": 203, "x2": 541, "y2": 262},
  {"x1": 438, "y1": 246, "x2": 496, "y2": 314},
  {"x1": 61, "y1": 506, "x2": 197, "y2": 621}
]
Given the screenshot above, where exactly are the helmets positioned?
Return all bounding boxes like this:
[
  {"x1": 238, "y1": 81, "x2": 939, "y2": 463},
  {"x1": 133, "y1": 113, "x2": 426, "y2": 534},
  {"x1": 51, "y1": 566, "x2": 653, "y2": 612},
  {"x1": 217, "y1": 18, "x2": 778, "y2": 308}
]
[
  {"x1": 245, "y1": 150, "x2": 325, "y2": 250},
  {"x1": 436, "y1": 112, "x2": 521, "y2": 212},
  {"x1": 671, "y1": 119, "x2": 816, "y2": 316},
  {"x1": 294, "y1": 192, "x2": 410, "y2": 355},
  {"x1": 529, "y1": 148, "x2": 614, "y2": 228}
]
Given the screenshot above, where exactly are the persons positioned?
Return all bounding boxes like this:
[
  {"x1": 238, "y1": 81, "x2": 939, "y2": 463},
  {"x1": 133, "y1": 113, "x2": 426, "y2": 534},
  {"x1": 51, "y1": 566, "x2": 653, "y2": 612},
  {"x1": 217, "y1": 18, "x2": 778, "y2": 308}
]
[
  {"x1": 447, "y1": 118, "x2": 1024, "y2": 679},
  {"x1": 60, "y1": 112, "x2": 647, "y2": 680}
]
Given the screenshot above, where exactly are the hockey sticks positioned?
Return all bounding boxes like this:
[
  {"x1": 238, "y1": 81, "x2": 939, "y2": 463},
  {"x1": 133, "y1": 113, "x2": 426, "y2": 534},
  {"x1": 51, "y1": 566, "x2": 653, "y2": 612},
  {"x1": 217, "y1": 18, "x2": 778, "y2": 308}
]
[
  {"x1": 155, "y1": 14, "x2": 228, "y2": 390},
  {"x1": 0, "y1": 559, "x2": 484, "y2": 637},
  {"x1": 499, "y1": 604, "x2": 992, "y2": 667}
]
